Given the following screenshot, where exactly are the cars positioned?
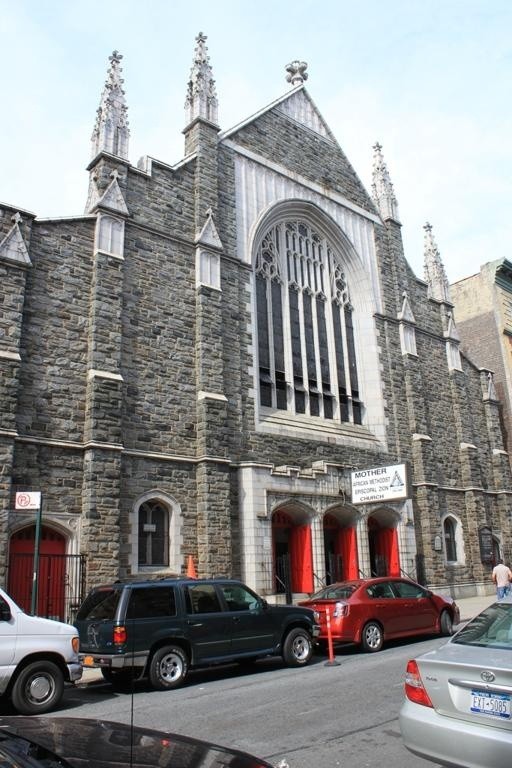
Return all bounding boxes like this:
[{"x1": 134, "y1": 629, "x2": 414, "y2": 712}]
[
  {"x1": 401, "y1": 596, "x2": 511, "y2": 767},
  {"x1": 297, "y1": 577, "x2": 462, "y2": 652},
  {"x1": 0, "y1": 715, "x2": 274, "y2": 768}
]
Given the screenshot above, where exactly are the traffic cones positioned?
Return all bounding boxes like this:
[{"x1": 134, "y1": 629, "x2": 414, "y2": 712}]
[{"x1": 186, "y1": 554, "x2": 197, "y2": 580}]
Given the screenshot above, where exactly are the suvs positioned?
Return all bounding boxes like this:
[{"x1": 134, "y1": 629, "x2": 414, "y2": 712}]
[
  {"x1": 72, "y1": 578, "x2": 321, "y2": 690},
  {"x1": 0, "y1": 588, "x2": 83, "y2": 715}
]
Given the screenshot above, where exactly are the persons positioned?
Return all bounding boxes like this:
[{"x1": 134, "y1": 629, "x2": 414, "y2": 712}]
[{"x1": 491, "y1": 559, "x2": 512, "y2": 600}]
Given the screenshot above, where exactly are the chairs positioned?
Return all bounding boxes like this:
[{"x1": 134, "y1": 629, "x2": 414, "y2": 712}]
[{"x1": 198, "y1": 597, "x2": 221, "y2": 613}]
